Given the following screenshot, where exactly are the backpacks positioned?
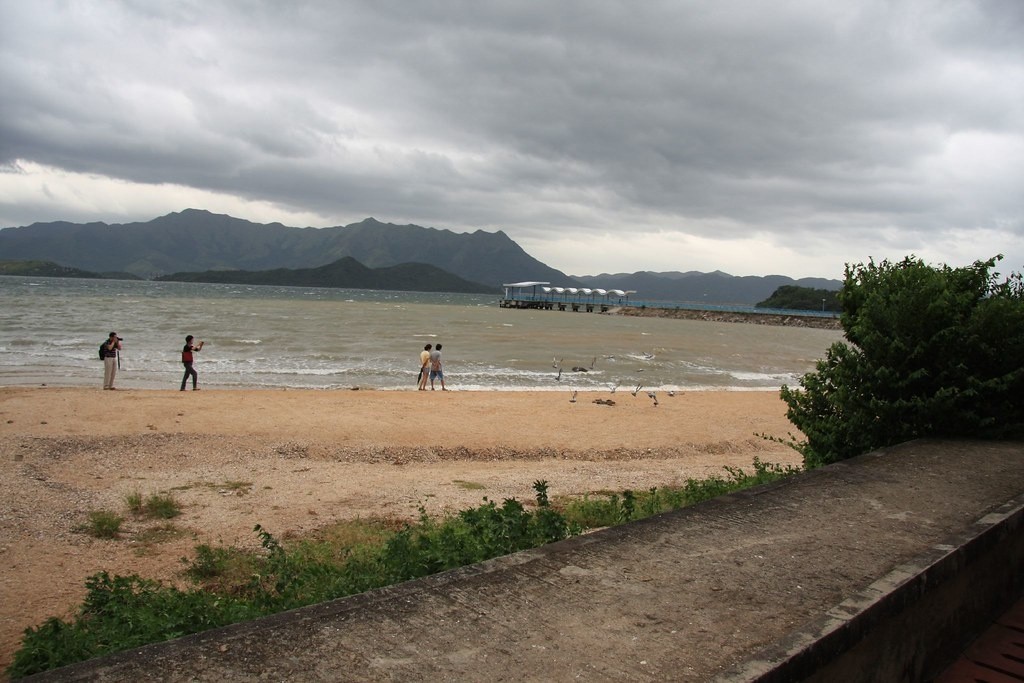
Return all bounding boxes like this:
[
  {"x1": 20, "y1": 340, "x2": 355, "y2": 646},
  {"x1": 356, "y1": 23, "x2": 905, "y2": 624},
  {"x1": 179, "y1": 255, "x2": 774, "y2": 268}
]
[{"x1": 99, "y1": 345, "x2": 104, "y2": 360}]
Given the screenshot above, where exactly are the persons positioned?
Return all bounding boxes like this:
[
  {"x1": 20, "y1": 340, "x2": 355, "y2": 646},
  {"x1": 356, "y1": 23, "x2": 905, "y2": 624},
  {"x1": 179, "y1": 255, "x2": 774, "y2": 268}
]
[
  {"x1": 430, "y1": 344, "x2": 448, "y2": 390},
  {"x1": 180, "y1": 335, "x2": 205, "y2": 391},
  {"x1": 99, "y1": 332, "x2": 123, "y2": 390},
  {"x1": 417, "y1": 344, "x2": 432, "y2": 391}
]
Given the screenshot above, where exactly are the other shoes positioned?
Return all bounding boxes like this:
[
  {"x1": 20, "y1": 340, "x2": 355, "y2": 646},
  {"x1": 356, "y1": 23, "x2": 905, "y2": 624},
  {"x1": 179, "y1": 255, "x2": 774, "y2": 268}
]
[
  {"x1": 179, "y1": 389, "x2": 187, "y2": 391},
  {"x1": 110, "y1": 387, "x2": 115, "y2": 390},
  {"x1": 418, "y1": 388, "x2": 427, "y2": 391},
  {"x1": 193, "y1": 388, "x2": 200, "y2": 391},
  {"x1": 431, "y1": 388, "x2": 434, "y2": 390},
  {"x1": 442, "y1": 388, "x2": 448, "y2": 391},
  {"x1": 103, "y1": 388, "x2": 110, "y2": 390}
]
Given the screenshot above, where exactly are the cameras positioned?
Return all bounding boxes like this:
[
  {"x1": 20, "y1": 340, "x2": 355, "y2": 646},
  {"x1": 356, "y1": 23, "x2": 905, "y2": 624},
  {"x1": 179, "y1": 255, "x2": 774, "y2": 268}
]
[{"x1": 117, "y1": 337, "x2": 123, "y2": 341}]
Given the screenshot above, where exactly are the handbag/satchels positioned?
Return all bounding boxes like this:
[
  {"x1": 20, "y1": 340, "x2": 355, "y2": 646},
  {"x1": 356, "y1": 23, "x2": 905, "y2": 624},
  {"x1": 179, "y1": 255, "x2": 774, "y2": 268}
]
[{"x1": 182, "y1": 351, "x2": 193, "y2": 362}]
